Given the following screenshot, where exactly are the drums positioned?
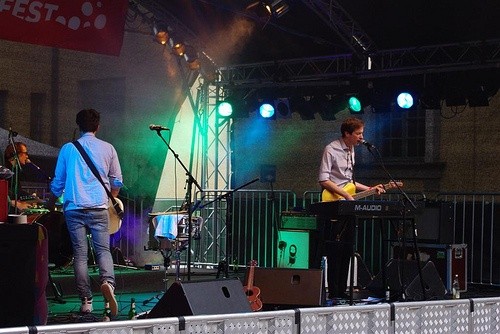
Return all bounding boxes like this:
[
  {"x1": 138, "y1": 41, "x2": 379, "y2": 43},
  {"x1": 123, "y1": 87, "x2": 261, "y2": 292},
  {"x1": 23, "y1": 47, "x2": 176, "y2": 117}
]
[{"x1": 20, "y1": 210, "x2": 75, "y2": 274}]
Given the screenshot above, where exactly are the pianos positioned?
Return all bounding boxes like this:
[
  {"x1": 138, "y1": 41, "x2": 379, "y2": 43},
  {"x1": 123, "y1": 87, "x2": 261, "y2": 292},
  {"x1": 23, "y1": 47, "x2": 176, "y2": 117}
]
[{"x1": 306, "y1": 200, "x2": 427, "y2": 306}]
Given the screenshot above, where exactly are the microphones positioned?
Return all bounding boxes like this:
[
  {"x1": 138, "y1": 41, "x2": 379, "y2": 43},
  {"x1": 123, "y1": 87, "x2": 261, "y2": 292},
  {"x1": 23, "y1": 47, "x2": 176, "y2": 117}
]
[
  {"x1": 149, "y1": 124, "x2": 170, "y2": 131},
  {"x1": 25, "y1": 158, "x2": 39, "y2": 170},
  {"x1": 359, "y1": 140, "x2": 377, "y2": 149}
]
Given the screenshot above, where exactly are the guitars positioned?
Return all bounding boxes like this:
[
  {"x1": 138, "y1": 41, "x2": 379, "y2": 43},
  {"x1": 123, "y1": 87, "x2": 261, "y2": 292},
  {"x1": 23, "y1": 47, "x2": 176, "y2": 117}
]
[
  {"x1": 243, "y1": 260, "x2": 262, "y2": 311},
  {"x1": 19, "y1": 195, "x2": 125, "y2": 235},
  {"x1": 321, "y1": 179, "x2": 404, "y2": 221}
]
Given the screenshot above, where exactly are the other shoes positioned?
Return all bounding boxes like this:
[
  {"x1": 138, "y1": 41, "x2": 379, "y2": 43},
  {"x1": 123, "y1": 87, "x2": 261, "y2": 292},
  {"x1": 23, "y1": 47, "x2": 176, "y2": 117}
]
[
  {"x1": 328, "y1": 290, "x2": 350, "y2": 299},
  {"x1": 101, "y1": 282, "x2": 117, "y2": 317},
  {"x1": 80, "y1": 296, "x2": 92, "y2": 313}
]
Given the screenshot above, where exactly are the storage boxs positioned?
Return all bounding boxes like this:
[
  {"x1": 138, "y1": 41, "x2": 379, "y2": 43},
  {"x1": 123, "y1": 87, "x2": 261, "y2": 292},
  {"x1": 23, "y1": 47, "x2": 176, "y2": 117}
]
[{"x1": 390, "y1": 238, "x2": 467, "y2": 295}]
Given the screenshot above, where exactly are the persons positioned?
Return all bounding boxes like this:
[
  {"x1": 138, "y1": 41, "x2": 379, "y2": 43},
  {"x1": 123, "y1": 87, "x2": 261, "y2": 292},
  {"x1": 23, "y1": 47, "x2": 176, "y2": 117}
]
[
  {"x1": 47, "y1": 108, "x2": 122, "y2": 318},
  {"x1": 2, "y1": 142, "x2": 34, "y2": 214},
  {"x1": 318, "y1": 118, "x2": 386, "y2": 303}
]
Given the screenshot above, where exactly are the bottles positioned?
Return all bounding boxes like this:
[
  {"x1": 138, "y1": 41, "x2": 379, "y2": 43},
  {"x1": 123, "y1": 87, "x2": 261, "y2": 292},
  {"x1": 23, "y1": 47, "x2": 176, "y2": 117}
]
[
  {"x1": 103, "y1": 298, "x2": 111, "y2": 322},
  {"x1": 129, "y1": 298, "x2": 137, "y2": 320},
  {"x1": 233, "y1": 257, "x2": 239, "y2": 273},
  {"x1": 452, "y1": 275, "x2": 460, "y2": 299},
  {"x1": 385, "y1": 284, "x2": 391, "y2": 303}
]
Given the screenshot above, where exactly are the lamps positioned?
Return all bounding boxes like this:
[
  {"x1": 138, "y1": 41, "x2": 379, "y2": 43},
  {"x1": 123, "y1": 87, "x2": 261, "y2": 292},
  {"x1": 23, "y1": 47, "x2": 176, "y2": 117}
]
[{"x1": 149, "y1": 15, "x2": 500, "y2": 121}]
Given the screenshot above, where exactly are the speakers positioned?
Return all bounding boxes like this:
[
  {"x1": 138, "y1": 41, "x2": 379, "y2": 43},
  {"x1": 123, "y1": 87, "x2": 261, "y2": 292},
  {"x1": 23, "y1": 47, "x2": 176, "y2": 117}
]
[
  {"x1": 367, "y1": 258, "x2": 448, "y2": 302},
  {"x1": 149, "y1": 279, "x2": 252, "y2": 320},
  {"x1": 244, "y1": 266, "x2": 325, "y2": 307}
]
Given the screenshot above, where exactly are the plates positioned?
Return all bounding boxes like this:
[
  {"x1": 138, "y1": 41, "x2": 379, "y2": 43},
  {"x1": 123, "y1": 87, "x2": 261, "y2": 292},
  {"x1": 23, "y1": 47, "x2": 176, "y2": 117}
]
[{"x1": 7, "y1": 214, "x2": 28, "y2": 224}]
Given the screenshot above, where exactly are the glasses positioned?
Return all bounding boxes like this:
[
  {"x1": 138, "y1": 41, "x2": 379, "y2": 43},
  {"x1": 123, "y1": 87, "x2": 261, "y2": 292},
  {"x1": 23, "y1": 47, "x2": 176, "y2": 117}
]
[{"x1": 18, "y1": 151, "x2": 28, "y2": 155}]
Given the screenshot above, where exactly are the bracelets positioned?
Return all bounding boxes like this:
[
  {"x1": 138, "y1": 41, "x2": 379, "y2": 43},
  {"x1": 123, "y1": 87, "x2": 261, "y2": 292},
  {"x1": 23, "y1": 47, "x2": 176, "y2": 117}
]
[{"x1": 369, "y1": 186, "x2": 372, "y2": 190}]
